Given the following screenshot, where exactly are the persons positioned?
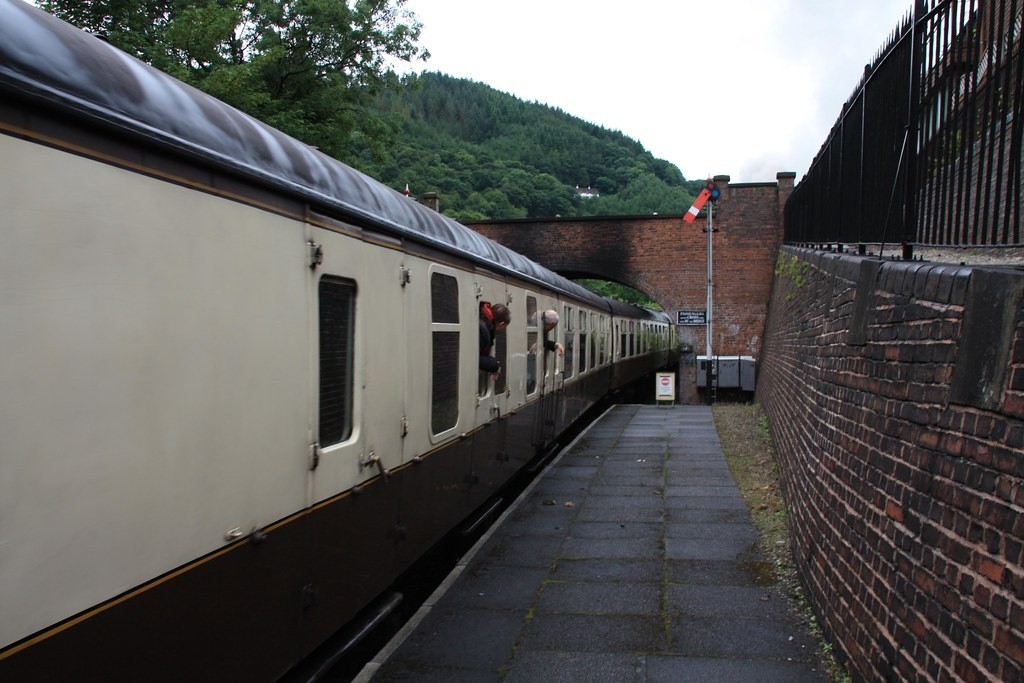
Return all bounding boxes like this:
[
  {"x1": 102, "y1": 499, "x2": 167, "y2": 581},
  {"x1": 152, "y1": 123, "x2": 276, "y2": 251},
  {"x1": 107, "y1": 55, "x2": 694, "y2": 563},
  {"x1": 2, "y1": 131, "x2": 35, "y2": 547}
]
[
  {"x1": 543, "y1": 311, "x2": 565, "y2": 357},
  {"x1": 479, "y1": 303, "x2": 511, "y2": 379},
  {"x1": 527, "y1": 310, "x2": 537, "y2": 352}
]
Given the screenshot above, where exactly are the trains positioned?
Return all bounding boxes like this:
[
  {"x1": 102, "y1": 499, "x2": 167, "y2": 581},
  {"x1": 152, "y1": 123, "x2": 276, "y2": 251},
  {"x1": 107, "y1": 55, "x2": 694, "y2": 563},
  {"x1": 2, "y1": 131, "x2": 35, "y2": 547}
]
[{"x1": 0, "y1": 0, "x2": 678, "y2": 683}]
